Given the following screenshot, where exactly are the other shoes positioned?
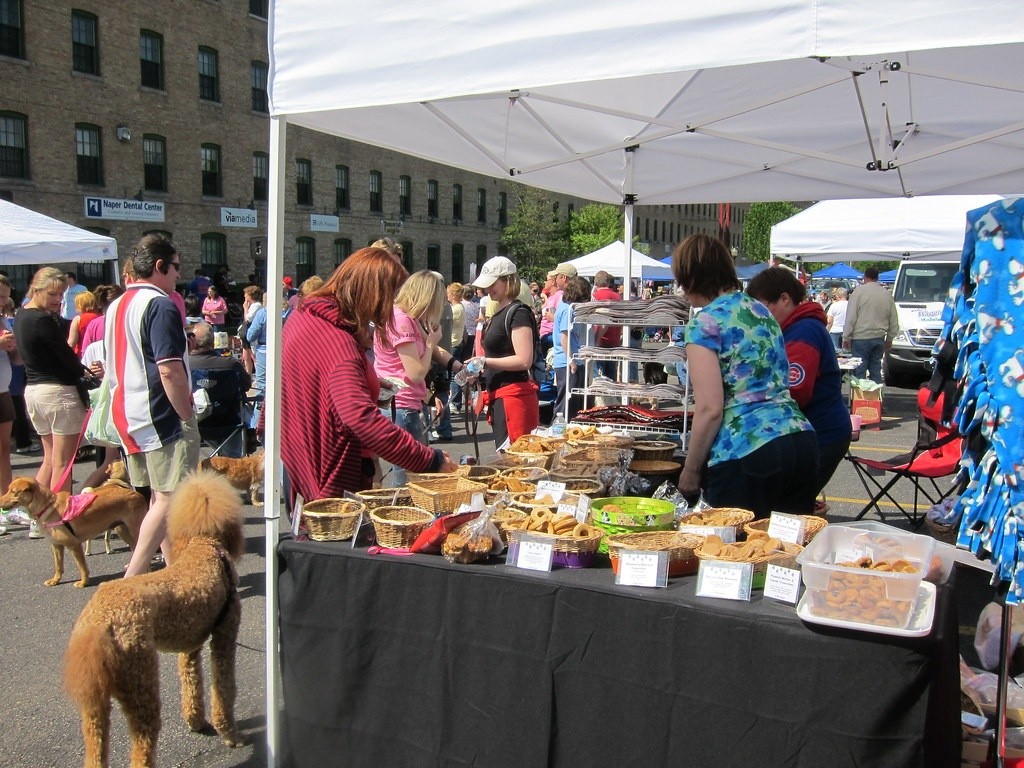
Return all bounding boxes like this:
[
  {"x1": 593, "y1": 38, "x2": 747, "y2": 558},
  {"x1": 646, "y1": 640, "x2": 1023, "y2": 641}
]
[
  {"x1": 428, "y1": 430, "x2": 433, "y2": 443},
  {"x1": 432, "y1": 430, "x2": 452, "y2": 440},
  {"x1": 449, "y1": 404, "x2": 460, "y2": 415}
]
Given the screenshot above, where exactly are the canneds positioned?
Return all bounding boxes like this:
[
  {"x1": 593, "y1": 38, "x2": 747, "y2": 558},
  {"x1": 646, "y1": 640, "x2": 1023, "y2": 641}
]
[
  {"x1": 2, "y1": 329, "x2": 13, "y2": 335},
  {"x1": 91, "y1": 360, "x2": 105, "y2": 379}
]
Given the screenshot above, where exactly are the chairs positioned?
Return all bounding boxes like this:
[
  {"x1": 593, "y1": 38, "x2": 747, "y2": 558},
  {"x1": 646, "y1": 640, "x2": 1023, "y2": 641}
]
[
  {"x1": 190, "y1": 368, "x2": 247, "y2": 460},
  {"x1": 843, "y1": 387, "x2": 964, "y2": 532}
]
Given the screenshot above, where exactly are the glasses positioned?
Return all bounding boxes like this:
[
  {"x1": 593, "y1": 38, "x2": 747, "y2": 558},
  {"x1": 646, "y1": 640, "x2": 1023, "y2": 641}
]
[
  {"x1": 155, "y1": 258, "x2": 180, "y2": 272},
  {"x1": 534, "y1": 290, "x2": 538, "y2": 292},
  {"x1": 545, "y1": 277, "x2": 554, "y2": 282}
]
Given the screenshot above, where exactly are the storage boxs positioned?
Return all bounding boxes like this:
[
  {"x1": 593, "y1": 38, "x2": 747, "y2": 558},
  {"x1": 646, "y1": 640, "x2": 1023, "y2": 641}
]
[
  {"x1": 796, "y1": 525, "x2": 937, "y2": 630},
  {"x1": 824, "y1": 520, "x2": 957, "y2": 584},
  {"x1": 852, "y1": 386, "x2": 880, "y2": 423}
]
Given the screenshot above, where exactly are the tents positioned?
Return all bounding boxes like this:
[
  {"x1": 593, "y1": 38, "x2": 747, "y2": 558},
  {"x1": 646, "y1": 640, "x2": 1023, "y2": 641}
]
[
  {"x1": 0, "y1": 199, "x2": 122, "y2": 286},
  {"x1": 877, "y1": 269, "x2": 899, "y2": 287},
  {"x1": 734, "y1": 261, "x2": 772, "y2": 293},
  {"x1": 811, "y1": 261, "x2": 865, "y2": 284},
  {"x1": 771, "y1": 195, "x2": 1002, "y2": 262},
  {"x1": 254, "y1": 0, "x2": 1024, "y2": 768},
  {"x1": 641, "y1": 256, "x2": 676, "y2": 287},
  {"x1": 564, "y1": 239, "x2": 671, "y2": 292}
]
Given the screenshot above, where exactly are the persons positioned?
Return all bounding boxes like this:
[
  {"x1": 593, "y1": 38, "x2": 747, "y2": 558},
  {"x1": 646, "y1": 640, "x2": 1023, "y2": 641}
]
[
  {"x1": 745, "y1": 267, "x2": 852, "y2": 499},
  {"x1": 670, "y1": 233, "x2": 821, "y2": 521},
  {"x1": 842, "y1": 268, "x2": 899, "y2": 413},
  {"x1": 0, "y1": 233, "x2": 702, "y2": 570},
  {"x1": 802, "y1": 287, "x2": 849, "y2": 347}
]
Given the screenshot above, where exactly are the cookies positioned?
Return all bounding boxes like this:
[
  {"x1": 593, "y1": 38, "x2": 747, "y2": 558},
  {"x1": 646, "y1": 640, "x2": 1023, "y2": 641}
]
[
  {"x1": 445, "y1": 506, "x2": 597, "y2": 564},
  {"x1": 690, "y1": 515, "x2": 781, "y2": 560},
  {"x1": 510, "y1": 425, "x2": 596, "y2": 453},
  {"x1": 811, "y1": 532, "x2": 916, "y2": 627},
  {"x1": 490, "y1": 470, "x2": 565, "y2": 505}
]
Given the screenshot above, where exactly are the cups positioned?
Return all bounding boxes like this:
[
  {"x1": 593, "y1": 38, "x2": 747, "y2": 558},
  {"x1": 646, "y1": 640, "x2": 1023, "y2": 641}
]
[{"x1": 849, "y1": 414, "x2": 862, "y2": 440}]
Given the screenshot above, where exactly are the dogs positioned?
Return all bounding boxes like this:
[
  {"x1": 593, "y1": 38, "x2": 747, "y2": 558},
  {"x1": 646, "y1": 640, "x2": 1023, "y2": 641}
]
[
  {"x1": 197, "y1": 450, "x2": 266, "y2": 508},
  {"x1": 65, "y1": 469, "x2": 251, "y2": 768},
  {"x1": 79, "y1": 460, "x2": 132, "y2": 557},
  {"x1": 0, "y1": 476, "x2": 150, "y2": 590}
]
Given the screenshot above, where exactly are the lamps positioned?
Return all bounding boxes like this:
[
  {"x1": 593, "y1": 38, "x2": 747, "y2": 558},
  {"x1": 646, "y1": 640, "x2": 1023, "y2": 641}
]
[
  {"x1": 117, "y1": 127, "x2": 131, "y2": 141},
  {"x1": 123, "y1": 187, "x2": 459, "y2": 227}
]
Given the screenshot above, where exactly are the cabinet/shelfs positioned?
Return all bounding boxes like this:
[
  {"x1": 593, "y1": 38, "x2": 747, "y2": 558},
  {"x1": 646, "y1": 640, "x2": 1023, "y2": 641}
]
[{"x1": 565, "y1": 303, "x2": 695, "y2": 452}]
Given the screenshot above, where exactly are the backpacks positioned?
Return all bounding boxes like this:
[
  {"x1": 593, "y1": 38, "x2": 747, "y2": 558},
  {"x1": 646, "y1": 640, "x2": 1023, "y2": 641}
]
[{"x1": 504, "y1": 303, "x2": 546, "y2": 382}]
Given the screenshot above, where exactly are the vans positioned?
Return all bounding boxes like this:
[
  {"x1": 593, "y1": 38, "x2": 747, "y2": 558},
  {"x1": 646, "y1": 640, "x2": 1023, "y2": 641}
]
[{"x1": 882, "y1": 261, "x2": 962, "y2": 386}]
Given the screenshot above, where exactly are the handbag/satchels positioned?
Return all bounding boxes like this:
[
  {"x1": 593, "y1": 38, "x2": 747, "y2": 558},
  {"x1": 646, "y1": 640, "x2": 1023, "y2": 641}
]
[{"x1": 84, "y1": 373, "x2": 123, "y2": 448}]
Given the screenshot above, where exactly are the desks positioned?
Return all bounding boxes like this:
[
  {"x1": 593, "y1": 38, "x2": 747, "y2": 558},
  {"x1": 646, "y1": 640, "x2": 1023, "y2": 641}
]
[{"x1": 274, "y1": 522, "x2": 962, "y2": 768}]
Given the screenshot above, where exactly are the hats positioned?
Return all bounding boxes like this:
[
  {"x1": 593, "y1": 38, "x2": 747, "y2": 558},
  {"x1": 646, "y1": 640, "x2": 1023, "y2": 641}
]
[
  {"x1": 284, "y1": 277, "x2": 293, "y2": 289},
  {"x1": 471, "y1": 256, "x2": 516, "y2": 289},
  {"x1": 548, "y1": 263, "x2": 577, "y2": 278}
]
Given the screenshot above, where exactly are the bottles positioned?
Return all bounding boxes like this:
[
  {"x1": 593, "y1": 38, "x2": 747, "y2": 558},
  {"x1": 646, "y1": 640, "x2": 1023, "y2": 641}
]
[
  {"x1": 552, "y1": 412, "x2": 566, "y2": 437},
  {"x1": 454, "y1": 357, "x2": 487, "y2": 386}
]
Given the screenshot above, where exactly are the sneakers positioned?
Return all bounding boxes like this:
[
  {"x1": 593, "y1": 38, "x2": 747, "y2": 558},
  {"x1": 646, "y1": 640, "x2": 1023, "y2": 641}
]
[
  {"x1": 17, "y1": 444, "x2": 40, "y2": 453},
  {"x1": 0, "y1": 526, "x2": 7, "y2": 535},
  {"x1": 29, "y1": 520, "x2": 45, "y2": 539},
  {"x1": 0, "y1": 506, "x2": 32, "y2": 527}
]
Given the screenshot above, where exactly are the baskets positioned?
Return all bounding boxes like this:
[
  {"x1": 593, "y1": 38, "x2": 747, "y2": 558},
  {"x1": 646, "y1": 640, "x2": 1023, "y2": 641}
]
[
  {"x1": 925, "y1": 516, "x2": 957, "y2": 545},
  {"x1": 301, "y1": 435, "x2": 830, "y2": 587}
]
